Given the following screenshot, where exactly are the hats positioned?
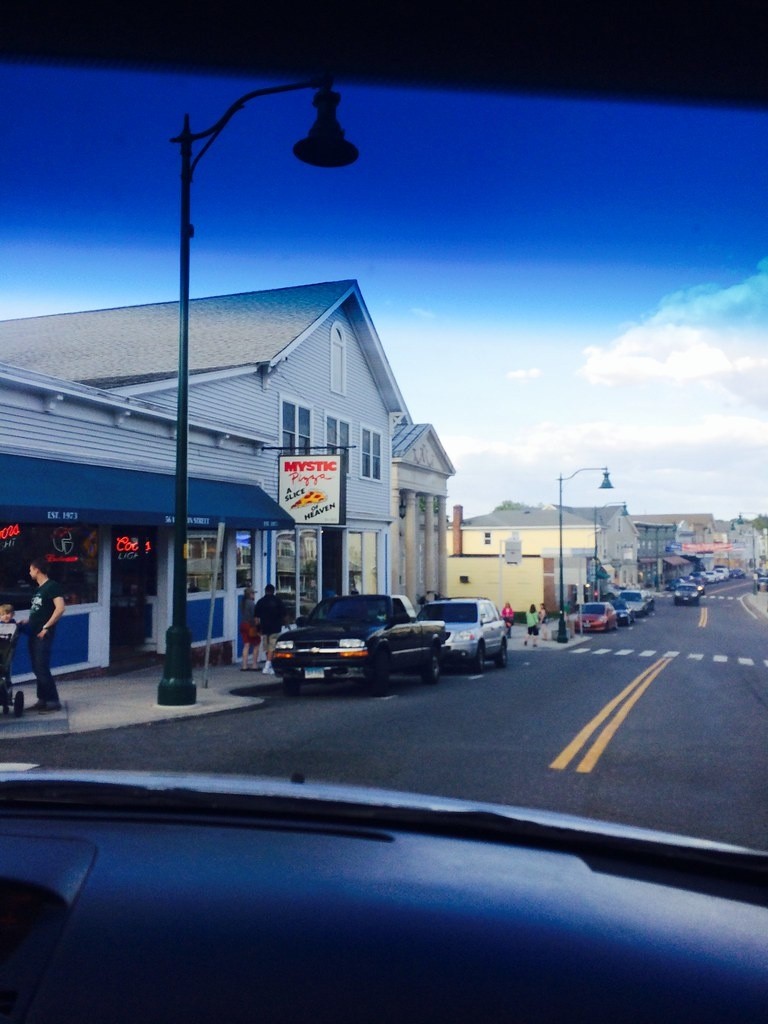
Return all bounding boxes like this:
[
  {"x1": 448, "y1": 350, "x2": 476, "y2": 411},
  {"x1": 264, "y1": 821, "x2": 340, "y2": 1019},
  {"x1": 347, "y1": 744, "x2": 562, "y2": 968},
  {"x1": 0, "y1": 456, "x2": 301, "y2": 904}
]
[{"x1": 244, "y1": 588, "x2": 258, "y2": 594}]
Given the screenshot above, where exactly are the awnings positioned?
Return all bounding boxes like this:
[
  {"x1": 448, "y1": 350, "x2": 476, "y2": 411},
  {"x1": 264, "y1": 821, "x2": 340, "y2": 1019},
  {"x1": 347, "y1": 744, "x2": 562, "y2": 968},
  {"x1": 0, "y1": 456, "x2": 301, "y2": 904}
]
[
  {"x1": 0, "y1": 452, "x2": 296, "y2": 530},
  {"x1": 663, "y1": 555, "x2": 689, "y2": 566}
]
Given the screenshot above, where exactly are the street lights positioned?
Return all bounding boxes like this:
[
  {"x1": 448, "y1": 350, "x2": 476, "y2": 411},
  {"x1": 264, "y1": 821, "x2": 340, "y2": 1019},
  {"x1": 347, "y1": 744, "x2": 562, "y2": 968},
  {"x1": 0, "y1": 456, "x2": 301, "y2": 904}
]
[
  {"x1": 594, "y1": 501, "x2": 629, "y2": 601},
  {"x1": 554, "y1": 465, "x2": 615, "y2": 644},
  {"x1": 731, "y1": 517, "x2": 759, "y2": 596},
  {"x1": 153, "y1": 78, "x2": 359, "y2": 707}
]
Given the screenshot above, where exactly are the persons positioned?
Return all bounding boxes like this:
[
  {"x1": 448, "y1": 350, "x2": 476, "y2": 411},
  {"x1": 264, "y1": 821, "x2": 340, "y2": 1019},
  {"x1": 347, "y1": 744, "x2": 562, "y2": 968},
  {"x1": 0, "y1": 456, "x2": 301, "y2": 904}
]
[
  {"x1": 564, "y1": 601, "x2": 571, "y2": 612},
  {"x1": 240, "y1": 589, "x2": 262, "y2": 671},
  {"x1": 188, "y1": 583, "x2": 200, "y2": 592},
  {"x1": 538, "y1": 603, "x2": 547, "y2": 640},
  {"x1": 502, "y1": 602, "x2": 514, "y2": 638},
  {"x1": 525, "y1": 604, "x2": 539, "y2": 647},
  {"x1": 16, "y1": 559, "x2": 65, "y2": 714},
  {"x1": 0, "y1": 603, "x2": 20, "y2": 687},
  {"x1": 254, "y1": 585, "x2": 289, "y2": 674}
]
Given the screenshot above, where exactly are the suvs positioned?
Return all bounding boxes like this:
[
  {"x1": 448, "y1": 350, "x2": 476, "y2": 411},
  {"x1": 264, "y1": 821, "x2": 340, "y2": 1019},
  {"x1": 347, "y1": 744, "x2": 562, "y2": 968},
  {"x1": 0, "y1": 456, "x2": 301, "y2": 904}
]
[{"x1": 417, "y1": 596, "x2": 509, "y2": 675}]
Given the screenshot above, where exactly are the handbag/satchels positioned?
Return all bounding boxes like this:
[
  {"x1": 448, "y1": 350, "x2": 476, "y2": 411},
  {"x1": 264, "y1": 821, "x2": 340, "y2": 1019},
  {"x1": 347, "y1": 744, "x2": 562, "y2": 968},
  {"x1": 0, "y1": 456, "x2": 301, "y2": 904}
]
[{"x1": 535, "y1": 622, "x2": 541, "y2": 630}]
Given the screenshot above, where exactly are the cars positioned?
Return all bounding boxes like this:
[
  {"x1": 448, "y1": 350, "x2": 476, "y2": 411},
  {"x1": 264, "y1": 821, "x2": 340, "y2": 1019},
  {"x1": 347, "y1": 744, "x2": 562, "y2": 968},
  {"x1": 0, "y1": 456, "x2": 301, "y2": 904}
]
[
  {"x1": 666, "y1": 568, "x2": 746, "y2": 591},
  {"x1": 617, "y1": 589, "x2": 655, "y2": 618},
  {"x1": 607, "y1": 600, "x2": 635, "y2": 626},
  {"x1": 674, "y1": 583, "x2": 700, "y2": 605},
  {"x1": 565, "y1": 602, "x2": 618, "y2": 632},
  {"x1": 688, "y1": 579, "x2": 704, "y2": 596}
]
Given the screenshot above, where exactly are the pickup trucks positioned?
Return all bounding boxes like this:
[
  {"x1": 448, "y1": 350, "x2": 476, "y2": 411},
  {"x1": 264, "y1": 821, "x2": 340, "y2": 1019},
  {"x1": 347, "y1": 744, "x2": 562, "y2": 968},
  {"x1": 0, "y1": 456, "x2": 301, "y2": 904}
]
[{"x1": 270, "y1": 593, "x2": 446, "y2": 694}]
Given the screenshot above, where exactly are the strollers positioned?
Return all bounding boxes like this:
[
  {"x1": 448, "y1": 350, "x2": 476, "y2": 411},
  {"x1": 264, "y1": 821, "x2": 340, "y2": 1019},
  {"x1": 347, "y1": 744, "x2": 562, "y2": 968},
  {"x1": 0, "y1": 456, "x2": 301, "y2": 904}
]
[{"x1": 0, "y1": 621, "x2": 25, "y2": 717}]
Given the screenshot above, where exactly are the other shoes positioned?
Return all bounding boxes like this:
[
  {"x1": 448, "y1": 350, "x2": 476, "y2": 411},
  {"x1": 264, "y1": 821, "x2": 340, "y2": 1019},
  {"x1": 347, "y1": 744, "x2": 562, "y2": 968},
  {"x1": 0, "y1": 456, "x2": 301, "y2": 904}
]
[
  {"x1": 262, "y1": 668, "x2": 269, "y2": 674},
  {"x1": 32, "y1": 699, "x2": 63, "y2": 715},
  {"x1": 241, "y1": 668, "x2": 249, "y2": 671},
  {"x1": 250, "y1": 668, "x2": 260, "y2": 671},
  {"x1": 268, "y1": 667, "x2": 275, "y2": 675}
]
